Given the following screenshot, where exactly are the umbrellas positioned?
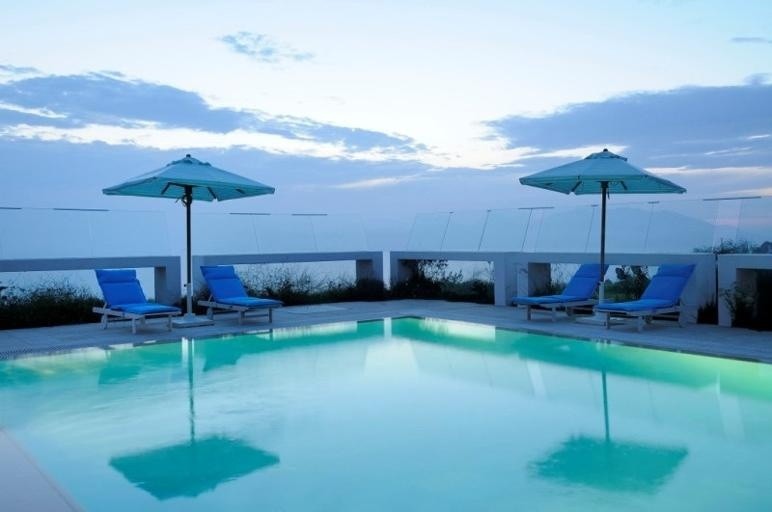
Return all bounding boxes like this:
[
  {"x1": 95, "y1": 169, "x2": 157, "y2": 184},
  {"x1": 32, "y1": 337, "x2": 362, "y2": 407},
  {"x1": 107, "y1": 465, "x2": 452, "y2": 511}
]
[
  {"x1": 103, "y1": 153, "x2": 278, "y2": 314},
  {"x1": 518, "y1": 148, "x2": 686, "y2": 304}
]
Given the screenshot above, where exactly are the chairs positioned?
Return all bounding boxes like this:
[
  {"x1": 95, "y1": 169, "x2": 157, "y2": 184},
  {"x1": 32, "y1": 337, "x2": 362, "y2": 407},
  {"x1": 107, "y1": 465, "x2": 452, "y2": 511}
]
[
  {"x1": 198, "y1": 265, "x2": 283, "y2": 326},
  {"x1": 511, "y1": 262, "x2": 696, "y2": 332},
  {"x1": 92, "y1": 269, "x2": 183, "y2": 334}
]
